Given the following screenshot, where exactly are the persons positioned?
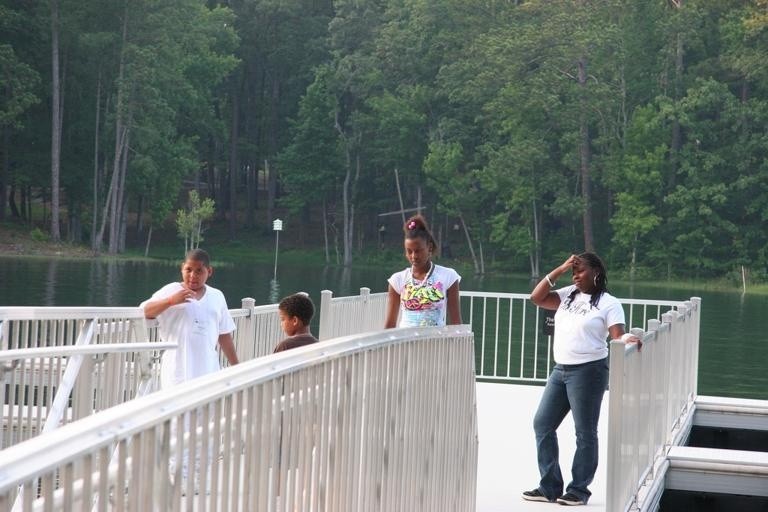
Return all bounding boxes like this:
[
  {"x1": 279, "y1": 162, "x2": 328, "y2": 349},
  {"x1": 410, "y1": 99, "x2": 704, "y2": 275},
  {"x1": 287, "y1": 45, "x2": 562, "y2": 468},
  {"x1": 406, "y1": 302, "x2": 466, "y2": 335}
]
[
  {"x1": 268, "y1": 291, "x2": 324, "y2": 502},
  {"x1": 517, "y1": 250, "x2": 644, "y2": 507},
  {"x1": 136, "y1": 247, "x2": 241, "y2": 496},
  {"x1": 380, "y1": 213, "x2": 464, "y2": 331}
]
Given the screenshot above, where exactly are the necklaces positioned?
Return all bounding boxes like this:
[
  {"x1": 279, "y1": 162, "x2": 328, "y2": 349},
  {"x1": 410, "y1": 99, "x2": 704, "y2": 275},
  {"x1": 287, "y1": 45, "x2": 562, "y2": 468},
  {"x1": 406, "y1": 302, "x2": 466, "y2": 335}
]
[{"x1": 409, "y1": 260, "x2": 433, "y2": 291}]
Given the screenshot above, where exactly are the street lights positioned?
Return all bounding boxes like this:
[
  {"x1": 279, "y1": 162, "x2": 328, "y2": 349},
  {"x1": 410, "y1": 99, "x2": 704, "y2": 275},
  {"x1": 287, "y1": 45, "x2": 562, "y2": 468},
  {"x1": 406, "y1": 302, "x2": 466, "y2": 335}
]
[
  {"x1": 379, "y1": 224, "x2": 385, "y2": 250},
  {"x1": 454, "y1": 222, "x2": 459, "y2": 260},
  {"x1": 272, "y1": 217, "x2": 283, "y2": 281}
]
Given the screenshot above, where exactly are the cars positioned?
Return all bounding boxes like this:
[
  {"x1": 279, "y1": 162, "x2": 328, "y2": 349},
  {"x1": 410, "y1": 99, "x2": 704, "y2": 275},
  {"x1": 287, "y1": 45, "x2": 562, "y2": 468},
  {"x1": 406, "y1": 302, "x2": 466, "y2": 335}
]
[{"x1": 198, "y1": 158, "x2": 269, "y2": 190}]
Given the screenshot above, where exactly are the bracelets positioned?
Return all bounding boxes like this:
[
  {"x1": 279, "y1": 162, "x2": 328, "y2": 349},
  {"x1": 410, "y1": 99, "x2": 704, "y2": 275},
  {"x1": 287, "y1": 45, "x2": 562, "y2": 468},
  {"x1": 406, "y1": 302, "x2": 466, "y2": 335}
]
[
  {"x1": 621, "y1": 331, "x2": 635, "y2": 343},
  {"x1": 544, "y1": 273, "x2": 556, "y2": 288},
  {"x1": 167, "y1": 294, "x2": 175, "y2": 306}
]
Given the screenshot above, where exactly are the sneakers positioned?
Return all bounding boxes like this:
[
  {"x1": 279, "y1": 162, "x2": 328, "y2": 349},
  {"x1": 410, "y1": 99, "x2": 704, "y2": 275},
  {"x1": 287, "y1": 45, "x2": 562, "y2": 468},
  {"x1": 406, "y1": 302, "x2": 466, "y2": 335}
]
[{"x1": 523, "y1": 488, "x2": 585, "y2": 506}]
